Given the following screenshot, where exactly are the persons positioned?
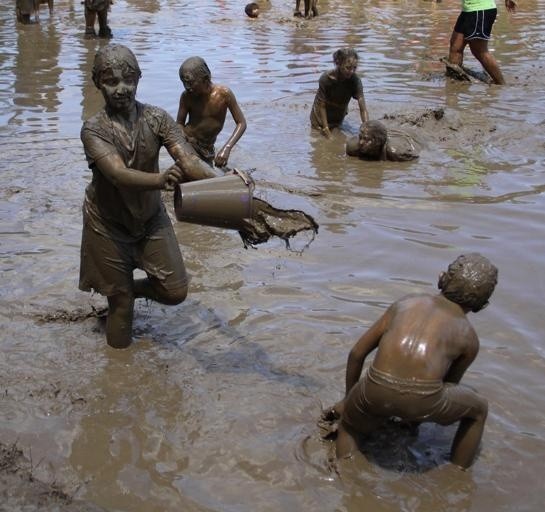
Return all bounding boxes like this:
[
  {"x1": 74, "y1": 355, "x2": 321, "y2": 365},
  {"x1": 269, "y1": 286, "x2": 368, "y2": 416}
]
[
  {"x1": 435, "y1": 0, "x2": 518, "y2": 86},
  {"x1": 15, "y1": 0, "x2": 54, "y2": 25},
  {"x1": 175, "y1": 56, "x2": 247, "y2": 170},
  {"x1": 293, "y1": 0, "x2": 319, "y2": 20},
  {"x1": 345, "y1": 108, "x2": 445, "y2": 162},
  {"x1": 83, "y1": 0, "x2": 114, "y2": 40},
  {"x1": 78, "y1": 42, "x2": 235, "y2": 349},
  {"x1": 329, "y1": 251, "x2": 499, "y2": 470},
  {"x1": 309, "y1": 47, "x2": 369, "y2": 141}
]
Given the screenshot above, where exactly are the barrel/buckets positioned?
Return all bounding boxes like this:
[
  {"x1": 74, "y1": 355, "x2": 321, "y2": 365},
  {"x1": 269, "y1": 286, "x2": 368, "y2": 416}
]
[{"x1": 174, "y1": 167, "x2": 255, "y2": 230}]
